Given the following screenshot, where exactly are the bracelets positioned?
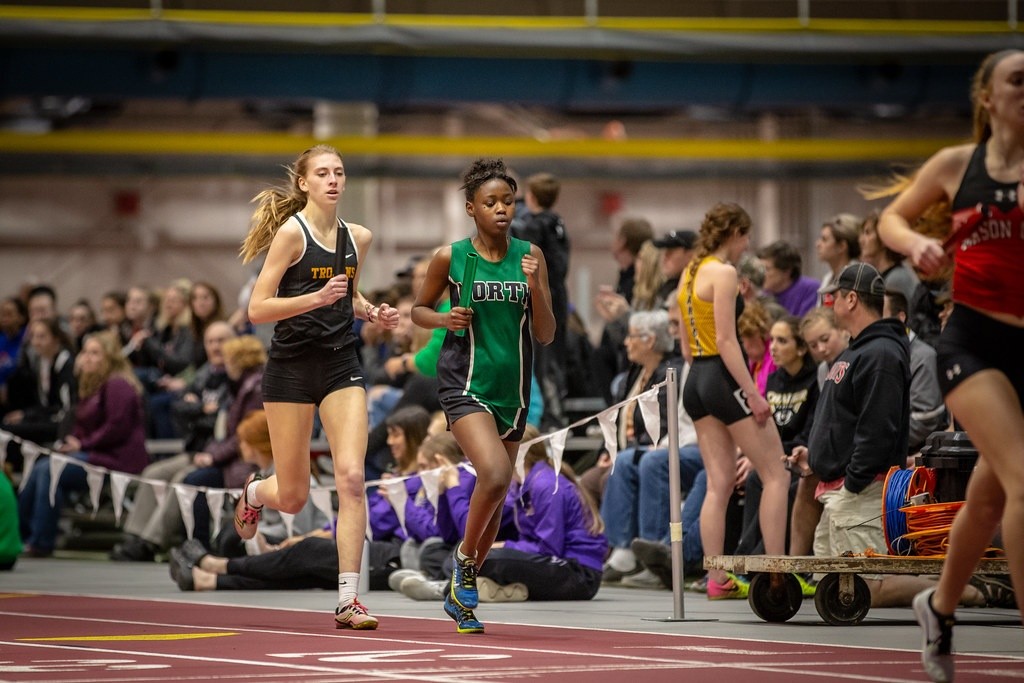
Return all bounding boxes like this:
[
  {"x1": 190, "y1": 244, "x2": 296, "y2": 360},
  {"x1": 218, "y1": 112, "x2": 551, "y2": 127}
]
[{"x1": 366, "y1": 305, "x2": 378, "y2": 324}]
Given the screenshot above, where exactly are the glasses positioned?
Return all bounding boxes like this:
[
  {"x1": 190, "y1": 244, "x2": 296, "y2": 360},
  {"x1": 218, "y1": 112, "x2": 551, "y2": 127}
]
[{"x1": 626, "y1": 333, "x2": 649, "y2": 340}]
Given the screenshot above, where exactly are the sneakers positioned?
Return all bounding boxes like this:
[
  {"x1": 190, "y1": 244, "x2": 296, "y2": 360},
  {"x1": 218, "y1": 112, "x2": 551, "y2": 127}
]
[
  {"x1": 794, "y1": 571, "x2": 818, "y2": 598},
  {"x1": 235, "y1": 473, "x2": 266, "y2": 540},
  {"x1": 706, "y1": 571, "x2": 751, "y2": 603},
  {"x1": 911, "y1": 584, "x2": 959, "y2": 682},
  {"x1": 443, "y1": 593, "x2": 485, "y2": 635},
  {"x1": 451, "y1": 538, "x2": 480, "y2": 610},
  {"x1": 333, "y1": 597, "x2": 380, "y2": 630}
]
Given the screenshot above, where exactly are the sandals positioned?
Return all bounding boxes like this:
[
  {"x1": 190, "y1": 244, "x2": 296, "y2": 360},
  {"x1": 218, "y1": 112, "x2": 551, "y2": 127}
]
[{"x1": 963, "y1": 572, "x2": 1020, "y2": 610}]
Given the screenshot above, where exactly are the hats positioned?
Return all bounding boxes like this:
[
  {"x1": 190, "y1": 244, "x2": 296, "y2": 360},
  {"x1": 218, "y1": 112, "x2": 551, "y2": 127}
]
[
  {"x1": 815, "y1": 263, "x2": 888, "y2": 298},
  {"x1": 650, "y1": 228, "x2": 698, "y2": 248}
]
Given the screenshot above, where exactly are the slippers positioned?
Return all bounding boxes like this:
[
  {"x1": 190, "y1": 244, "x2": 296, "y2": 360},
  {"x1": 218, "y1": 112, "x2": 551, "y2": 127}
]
[{"x1": 170, "y1": 536, "x2": 209, "y2": 591}]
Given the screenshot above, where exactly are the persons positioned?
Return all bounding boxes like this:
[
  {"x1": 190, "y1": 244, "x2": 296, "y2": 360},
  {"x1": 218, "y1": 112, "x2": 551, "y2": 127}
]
[
  {"x1": 234, "y1": 145, "x2": 399, "y2": 628},
  {"x1": 676, "y1": 202, "x2": 816, "y2": 599},
  {"x1": 878, "y1": 50, "x2": 1023, "y2": 683},
  {"x1": 510, "y1": 174, "x2": 569, "y2": 430},
  {"x1": 411, "y1": 160, "x2": 557, "y2": 632},
  {"x1": 580, "y1": 219, "x2": 701, "y2": 585},
  {"x1": 0, "y1": 253, "x2": 605, "y2": 592},
  {"x1": 633, "y1": 215, "x2": 1007, "y2": 604}
]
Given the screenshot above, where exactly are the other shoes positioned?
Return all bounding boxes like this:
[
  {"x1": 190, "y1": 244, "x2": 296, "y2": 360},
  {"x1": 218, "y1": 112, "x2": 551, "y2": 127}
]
[
  {"x1": 387, "y1": 569, "x2": 530, "y2": 602},
  {"x1": 687, "y1": 576, "x2": 709, "y2": 592},
  {"x1": 110, "y1": 539, "x2": 154, "y2": 567},
  {"x1": 602, "y1": 559, "x2": 646, "y2": 583},
  {"x1": 629, "y1": 539, "x2": 675, "y2": 592},
  {"x1": 18, "y1": 541, "x2": 50, "y2": 558}
]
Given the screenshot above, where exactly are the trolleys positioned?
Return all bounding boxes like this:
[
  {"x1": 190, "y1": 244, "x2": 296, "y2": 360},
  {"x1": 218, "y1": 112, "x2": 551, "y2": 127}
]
[{"x1": 703, "y1": 431, "x2": 1010, "y2": 626}]
